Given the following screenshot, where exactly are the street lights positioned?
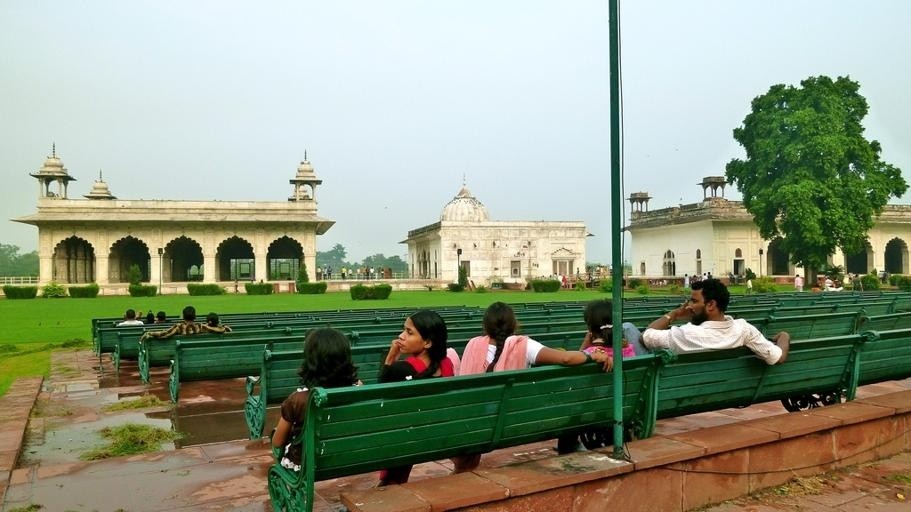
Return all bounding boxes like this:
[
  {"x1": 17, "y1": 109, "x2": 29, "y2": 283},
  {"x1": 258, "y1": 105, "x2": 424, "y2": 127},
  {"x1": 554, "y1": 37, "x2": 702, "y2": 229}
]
[
  {"x1": 457, "y1": 248, "x2": 462, "y2": 267},
  {"x1": 157, "y1": 247, "x2": 163, "y2": 294},
  {"x1": 759, "y1": 248, "x2": 763, "y2": 277}
]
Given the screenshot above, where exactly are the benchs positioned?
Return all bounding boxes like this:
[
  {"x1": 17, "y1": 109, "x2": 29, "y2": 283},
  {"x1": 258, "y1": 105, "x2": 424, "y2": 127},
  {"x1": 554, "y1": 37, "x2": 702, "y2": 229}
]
[{"x1": 89, "y1": 286, "x2": 911, "y2": 512}]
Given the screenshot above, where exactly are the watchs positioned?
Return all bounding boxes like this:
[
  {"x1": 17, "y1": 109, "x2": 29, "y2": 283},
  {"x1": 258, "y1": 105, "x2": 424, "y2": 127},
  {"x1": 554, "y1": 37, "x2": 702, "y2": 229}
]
[{"x1": 664, "y1": 314, "x2": 673, "y2": 328}]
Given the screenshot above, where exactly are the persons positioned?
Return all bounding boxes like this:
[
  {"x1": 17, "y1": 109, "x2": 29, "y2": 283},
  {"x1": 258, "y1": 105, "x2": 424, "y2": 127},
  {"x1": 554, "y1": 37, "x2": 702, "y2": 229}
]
[
  {"x1": 140, "y1": 307, "x2": 232, "y2": 343},
  {"x1": 316, "y1": 265, "x2": 392, "y2": 281},
  {"x1": 729, "y1": 273, "x2": 752, "y2": 295},
  {"x1": 684, "y1": 271, "x2": 712, "y2": 289},
  {"x1": 622, "y1": 279, "x2": 791, "y2": 366},
  {"x1": 116, "y1": 309, "x2": 171, "y2": 327},
  {"x1": 794, "y1": 272, "x2": 864, "y2": 293},
  {"x1": 554, "y1": 265, "x2": 607, "y2": 291},
  {"x1": 235, "y1": 279, "x2": 240, "y2": 294},
  {"x1": 649, "y1": 278, "x2": 668, "y2": 288},
  {"x1": 446, "y1": 301, "x2": 614, "y2": 376},
  {"x1": 272, "y1": 328, "x2": 364, "y2": 476},
  {"x1": 373, "y1": 310, "x2": 481, "y2": 489},
  {"x1": 205, "y1": 312, "x2": 227, "y2": 332},
  {"x1": 558, "y1": 299, "x2": 637, "y2": 458},
  {"x1": 878, "y1": 270, "x2": 890, "y2": 285}
]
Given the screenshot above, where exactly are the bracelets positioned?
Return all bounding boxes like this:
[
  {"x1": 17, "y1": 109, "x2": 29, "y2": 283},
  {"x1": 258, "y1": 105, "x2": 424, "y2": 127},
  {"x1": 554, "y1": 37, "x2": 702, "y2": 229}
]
[{"x1": 581, "y1": 350, "x2": 592, "y2": 363}]
